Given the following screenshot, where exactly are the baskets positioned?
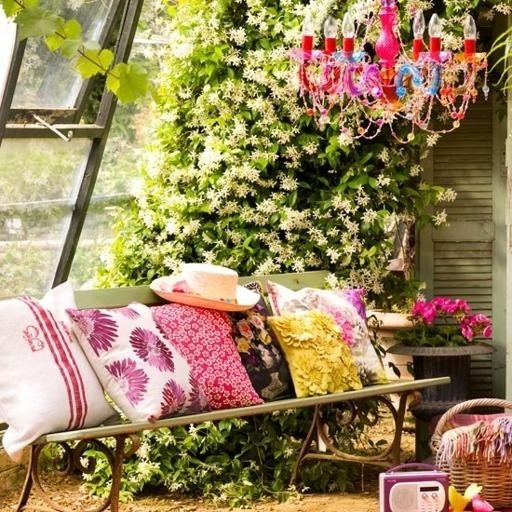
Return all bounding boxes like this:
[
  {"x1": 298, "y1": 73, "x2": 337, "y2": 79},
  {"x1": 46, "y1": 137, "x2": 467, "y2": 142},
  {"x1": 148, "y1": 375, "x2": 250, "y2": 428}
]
[{"x1": 428, "y1": 398, "x2": 512, "y2": 509}]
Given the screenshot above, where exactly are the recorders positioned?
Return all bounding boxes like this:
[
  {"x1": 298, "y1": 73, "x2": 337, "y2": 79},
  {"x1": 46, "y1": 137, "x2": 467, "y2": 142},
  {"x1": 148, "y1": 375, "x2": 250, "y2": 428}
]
[{"x1": 378, "y1": 462, "x2": 450, "y2": 512}]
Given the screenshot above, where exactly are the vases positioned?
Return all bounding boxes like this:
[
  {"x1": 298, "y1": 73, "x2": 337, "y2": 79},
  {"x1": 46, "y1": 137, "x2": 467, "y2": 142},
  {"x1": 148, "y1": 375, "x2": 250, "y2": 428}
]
[{"x1": 384, "y1": 342, "x2": 496, "y2": 400}]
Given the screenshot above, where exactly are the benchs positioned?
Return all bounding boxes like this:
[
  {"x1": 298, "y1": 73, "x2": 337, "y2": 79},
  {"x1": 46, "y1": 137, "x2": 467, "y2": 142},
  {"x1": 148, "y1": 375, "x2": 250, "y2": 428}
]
[{"x1": 1, "y1": 268, "x2": 453, "y2": 511}]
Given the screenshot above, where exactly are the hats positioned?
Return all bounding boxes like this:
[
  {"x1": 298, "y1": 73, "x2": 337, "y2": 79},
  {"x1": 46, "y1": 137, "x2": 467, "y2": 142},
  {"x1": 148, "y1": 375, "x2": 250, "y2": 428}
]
[{"x1": 149, "y1": 262, "x2": 260, "y2": 312}]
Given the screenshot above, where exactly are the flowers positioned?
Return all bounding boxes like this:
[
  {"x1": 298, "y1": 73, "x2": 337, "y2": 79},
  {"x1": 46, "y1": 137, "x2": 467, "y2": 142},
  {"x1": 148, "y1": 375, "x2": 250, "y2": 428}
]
[{"x1": 398, "y1": 295, "x2": 492, "y2": 346}]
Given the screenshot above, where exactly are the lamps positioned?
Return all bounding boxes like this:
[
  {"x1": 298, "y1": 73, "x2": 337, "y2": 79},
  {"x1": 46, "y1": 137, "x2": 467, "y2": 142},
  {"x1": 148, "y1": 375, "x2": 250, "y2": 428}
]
[{"x1": 286, "y1": 1, "x2": 491, "y2": 145}]
